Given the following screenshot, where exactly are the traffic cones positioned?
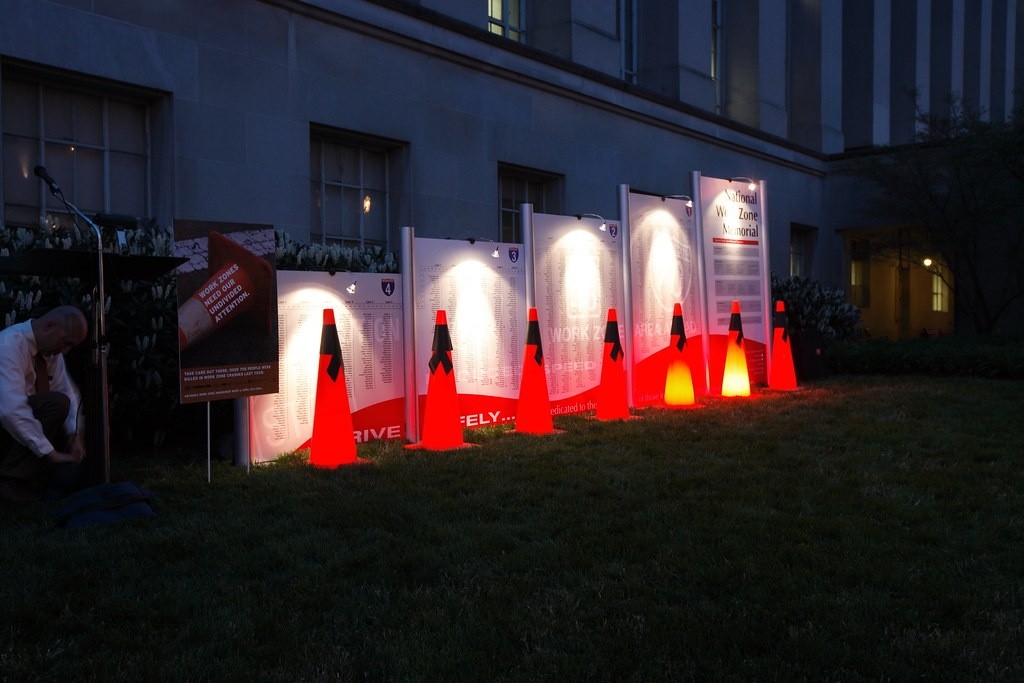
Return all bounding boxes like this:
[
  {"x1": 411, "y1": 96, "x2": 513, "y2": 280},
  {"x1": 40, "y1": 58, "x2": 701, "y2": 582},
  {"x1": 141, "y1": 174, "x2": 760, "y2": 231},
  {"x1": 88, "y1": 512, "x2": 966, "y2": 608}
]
[
  {"x1": 584, "y1": 309, "x2": 645, "y2": 424},
  {"x1": 306, "y1": 309, "x2": 361, "y2": 478},
  {"x1": 502, "y1": 309, "x2": 566, "y2": 438},
  {"x1": 661, "y1": 303, "x2": 700, "y2": 413},
  {"x1": 401, "y1": 310, "x2": 480, "y2": 454},
  {"x1": 179, "y1": 231, "x2": 273, "y2": 353},
  {"x1": 715, "y1": 303, "x2": 754, "y2": 402},
  {"x1": 769, "y1": 299, "x2": 803, "y2": 392}
]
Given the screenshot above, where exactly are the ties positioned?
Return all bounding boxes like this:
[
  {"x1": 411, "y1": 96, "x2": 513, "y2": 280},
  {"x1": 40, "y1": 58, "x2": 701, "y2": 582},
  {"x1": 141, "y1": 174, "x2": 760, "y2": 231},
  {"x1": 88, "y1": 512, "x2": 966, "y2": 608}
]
[{"x1": 35, "y1": 353, "x2": 49, "y2": 393}]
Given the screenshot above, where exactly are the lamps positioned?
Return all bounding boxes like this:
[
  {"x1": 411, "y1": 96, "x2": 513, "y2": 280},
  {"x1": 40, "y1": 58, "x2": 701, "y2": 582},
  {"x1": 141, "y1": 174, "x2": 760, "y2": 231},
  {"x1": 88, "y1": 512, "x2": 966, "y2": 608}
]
[
  {"x1": 468, "y1": 238, "x2": 499, "y2": 258},
  {"x1": 93, "y1": 213, "x2": 137, "y2": 240},
  {"x1": 728, "y1": 177, "x2": 756, "y2": 191},
  {"x1": 329, "y1": 266, "x2": 357, "y2": 293},
  {"x1": 576, "y1": 213, "x2": 606, "y2": 232},
  {"x1": 662, "y1": 194, "x2": 693, "y2": 207}
]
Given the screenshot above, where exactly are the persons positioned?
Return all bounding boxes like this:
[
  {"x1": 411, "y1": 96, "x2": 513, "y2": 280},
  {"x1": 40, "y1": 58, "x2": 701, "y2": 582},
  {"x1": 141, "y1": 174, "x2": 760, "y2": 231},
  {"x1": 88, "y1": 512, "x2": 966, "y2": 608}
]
[{"x1": 0, "y1": 305, "x2": 88, "y2": 489}]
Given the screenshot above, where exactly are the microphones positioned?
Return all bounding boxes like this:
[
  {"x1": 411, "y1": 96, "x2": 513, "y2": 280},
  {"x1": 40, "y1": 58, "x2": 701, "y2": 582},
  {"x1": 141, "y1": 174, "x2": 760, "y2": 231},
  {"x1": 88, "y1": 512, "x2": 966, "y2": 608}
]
[{"x1": 34, "y1": 165, "x2": 61, "y2": 194}]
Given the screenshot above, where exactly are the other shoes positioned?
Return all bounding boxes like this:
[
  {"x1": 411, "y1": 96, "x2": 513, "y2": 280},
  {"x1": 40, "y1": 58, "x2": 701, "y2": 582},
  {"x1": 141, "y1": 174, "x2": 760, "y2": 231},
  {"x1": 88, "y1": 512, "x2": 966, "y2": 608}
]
[{"x1": 0, "y1": 477, "x2": 25, "y2": 501}]
